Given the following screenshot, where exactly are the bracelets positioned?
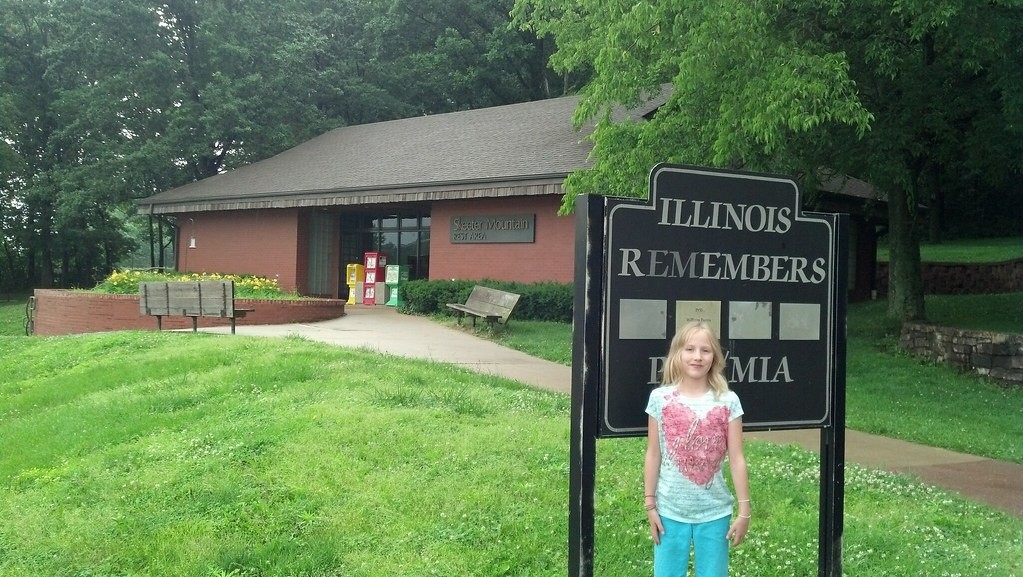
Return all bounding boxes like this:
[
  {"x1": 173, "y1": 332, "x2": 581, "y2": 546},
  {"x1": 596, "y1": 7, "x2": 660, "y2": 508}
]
[
  {"x1": 738, "y1": 514, "x2": 751, "y2": 519},
  {"x1": 737, "y1": 499, "x2": 750, "y2": 502},
  {"x1": 645, "y1": 503, "x2": 656, "y2": 511},
  {"x1": 644, "y1": 495, "x2": 656, "y2": 498}
]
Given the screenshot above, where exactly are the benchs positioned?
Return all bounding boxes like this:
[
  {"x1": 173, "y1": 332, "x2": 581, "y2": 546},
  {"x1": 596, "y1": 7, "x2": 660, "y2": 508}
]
[
  {"x1": 446, "y1": 284, "x2": 522, "y2": 335},
  {"x1": 137, "y1": 279, "x2": 255, "y2": 335}
]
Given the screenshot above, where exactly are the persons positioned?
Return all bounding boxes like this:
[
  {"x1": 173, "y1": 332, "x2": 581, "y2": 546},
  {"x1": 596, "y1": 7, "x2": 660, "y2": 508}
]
[{"x1": 643, "y1": 320, "x2": 752, "y2": 577}]
